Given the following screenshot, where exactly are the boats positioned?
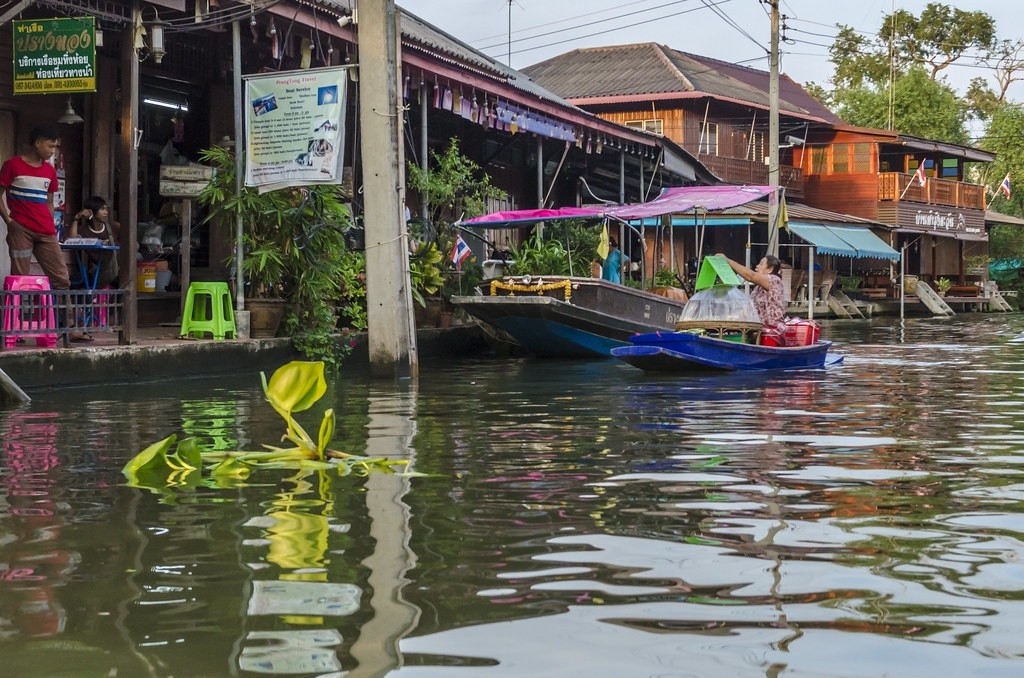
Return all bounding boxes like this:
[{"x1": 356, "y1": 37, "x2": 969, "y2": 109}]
[
  {"x1": 609, "y1": 332, "x2": 832, "y2": 375},
  {"x1": 448, "y1": 184, "x2": 780, "y2": 361}
]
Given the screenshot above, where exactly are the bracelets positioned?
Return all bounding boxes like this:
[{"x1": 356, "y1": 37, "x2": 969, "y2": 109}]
[{"x1": 73, "y1": 217, "x2": 79, "y2": 222}]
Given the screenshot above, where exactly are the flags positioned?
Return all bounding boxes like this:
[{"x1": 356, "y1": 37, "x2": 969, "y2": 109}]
[
  {"x1": 449, "y1": 234, "x2": 471, "y2": 272},
  {"x1": 916, "y1": 159, "x2": 926, "y2": 188},
  {"x1": 1000, "y1": 173, "x2": 1010, "y2": 200}
]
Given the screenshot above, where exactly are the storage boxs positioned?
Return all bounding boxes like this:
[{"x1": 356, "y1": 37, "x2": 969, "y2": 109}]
[
  {"x1": 780, "y1": 319, "x2": 821, "y2": 347},
  {"x1": 711, "y1": 333, "x2": 749, "y2": 343}
]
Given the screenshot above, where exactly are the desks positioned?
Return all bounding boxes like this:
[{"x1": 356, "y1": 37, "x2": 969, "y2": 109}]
[{"x1": 57, "y1": 245, "x2": 122, "y2": 330}]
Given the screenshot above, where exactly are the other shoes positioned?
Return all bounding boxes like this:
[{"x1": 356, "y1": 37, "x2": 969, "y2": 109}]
[
  {"x1": 17, "y1": 338, "x2": 25, "y2": 343},
  {"x1": 71, "y1": 331, "x2": 93, "y2": 342}
]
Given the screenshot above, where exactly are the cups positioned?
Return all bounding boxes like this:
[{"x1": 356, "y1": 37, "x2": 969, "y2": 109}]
[{"x1": 761, "y1": 334, "x2": 781, "y2": 347}]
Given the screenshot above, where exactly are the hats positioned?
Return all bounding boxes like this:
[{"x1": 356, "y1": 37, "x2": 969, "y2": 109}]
[{"x1": 84, "y1": 196, "x2": 106, "y2": 214}]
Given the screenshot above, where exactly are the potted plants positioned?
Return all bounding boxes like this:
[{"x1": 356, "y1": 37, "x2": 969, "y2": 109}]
[
  {"x1": 223, "y1": 234, "x2": 287, "y2": 339},
  {"x1": 934, "y1": 277, "x2": 951, "y2": 297},
  {"x1": 836, "y1": 276, "x2": 860, "y2": 291},
  {"x1": 407, "y1": 242, "x2": 453, "y2": 328}
]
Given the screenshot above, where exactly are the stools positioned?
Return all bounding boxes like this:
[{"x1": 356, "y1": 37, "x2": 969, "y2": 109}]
[
  {"x1": 77, "y1": 284, "x2": 112, "y2": 327},
  {"x1": 178, "y1": 282, "x2": 237, "y2": 340},
  {"x1": 4, "y1": 275, "x2": 58, "y2": 348}
]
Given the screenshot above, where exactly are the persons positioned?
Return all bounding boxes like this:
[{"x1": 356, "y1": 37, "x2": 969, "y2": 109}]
[
  {"x1": 595, "y1": 236, "x2": 630, "y2": 285},
  {"x1": 715, "y1": 253, "x2": 785, "y2": 326},
  {"x1": 68, "y1": 196, "x2": 120, "y2": 325},
  {"x1": 780, "y1": 256, "x2": 823, "y2": 271},
  {"x1": 0, "y1": 126, "x2": 94, "y2": 346}
]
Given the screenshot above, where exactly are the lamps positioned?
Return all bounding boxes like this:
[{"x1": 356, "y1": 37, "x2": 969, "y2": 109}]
[
  {"x1": 132, "y1": 5, "x2": 172, "y2": 63},
  {"x1": 57, "y1": 102, "x2": 85, "y2": 125},
  {"x1": 249, "y1": 6, "x2": 665, "y2": 166}
]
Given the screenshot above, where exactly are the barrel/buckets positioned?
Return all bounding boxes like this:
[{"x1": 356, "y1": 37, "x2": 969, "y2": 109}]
[{"x1": 137, "y1": 263, "x2": 157, "y2": 292}]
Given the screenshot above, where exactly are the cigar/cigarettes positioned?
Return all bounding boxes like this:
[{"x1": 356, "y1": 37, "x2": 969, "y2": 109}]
[{"x1": 89, "y1": 214, "x2": 93, "y2": 219}]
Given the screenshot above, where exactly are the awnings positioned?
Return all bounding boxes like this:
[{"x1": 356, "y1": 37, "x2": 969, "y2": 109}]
[{"x1": 787, "y1": 222, "x2": 902, "y2": 261}]
